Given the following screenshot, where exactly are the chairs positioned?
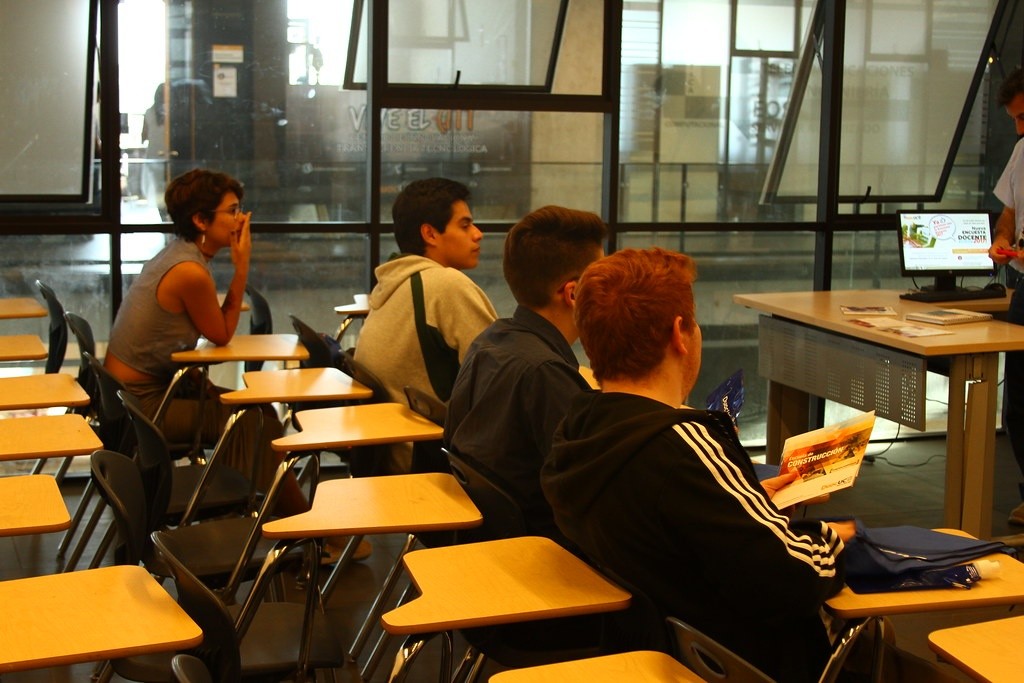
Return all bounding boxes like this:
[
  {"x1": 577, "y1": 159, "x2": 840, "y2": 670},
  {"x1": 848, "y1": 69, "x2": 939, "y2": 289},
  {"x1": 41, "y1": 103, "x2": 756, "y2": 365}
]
[{"x1": 1, "y1": 278, "x2": 1024, "y2": 683}]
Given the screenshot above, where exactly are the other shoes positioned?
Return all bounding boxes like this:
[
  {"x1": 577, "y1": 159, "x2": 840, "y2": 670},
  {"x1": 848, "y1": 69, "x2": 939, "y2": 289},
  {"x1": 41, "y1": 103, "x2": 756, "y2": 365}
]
[
  {"x1": 307, "y1": 537, "x2": 373, "y2": 565},
  {"x1": 1008, "y1": 501, "x2": 1024, "y2": 524}
]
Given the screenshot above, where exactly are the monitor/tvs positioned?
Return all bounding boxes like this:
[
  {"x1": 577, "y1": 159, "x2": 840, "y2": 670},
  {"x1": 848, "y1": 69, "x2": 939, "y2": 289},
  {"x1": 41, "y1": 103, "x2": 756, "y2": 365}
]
[{"x1": 896, "y1": 210, "x2": 997, "y2": 287}]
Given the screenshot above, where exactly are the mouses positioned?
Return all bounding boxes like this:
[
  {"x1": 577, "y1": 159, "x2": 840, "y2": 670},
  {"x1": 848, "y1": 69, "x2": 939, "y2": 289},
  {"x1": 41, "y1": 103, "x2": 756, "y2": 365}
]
[{"x1": 985, "y1": 283, "x2": 1005, "y2": 293}]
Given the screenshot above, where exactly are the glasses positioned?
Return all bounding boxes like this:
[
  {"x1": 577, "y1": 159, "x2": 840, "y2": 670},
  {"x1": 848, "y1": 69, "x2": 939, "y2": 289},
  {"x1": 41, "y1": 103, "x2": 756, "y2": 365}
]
[{"x1": 201, "y1": 203, "x2": 244, "y2": 220}]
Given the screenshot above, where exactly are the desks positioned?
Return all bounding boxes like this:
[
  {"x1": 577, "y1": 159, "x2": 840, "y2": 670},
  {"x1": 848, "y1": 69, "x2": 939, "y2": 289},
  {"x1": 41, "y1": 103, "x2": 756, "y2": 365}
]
[{"x1": 733, "y1": 288, "x2": 1024, "y2": 541}]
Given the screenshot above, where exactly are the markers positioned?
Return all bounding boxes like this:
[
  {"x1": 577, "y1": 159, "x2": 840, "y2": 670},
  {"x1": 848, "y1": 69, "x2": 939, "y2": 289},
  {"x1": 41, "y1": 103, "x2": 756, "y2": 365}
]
[{"x1": 997, "y1": 249, "x2": 1020, "y2": 257}]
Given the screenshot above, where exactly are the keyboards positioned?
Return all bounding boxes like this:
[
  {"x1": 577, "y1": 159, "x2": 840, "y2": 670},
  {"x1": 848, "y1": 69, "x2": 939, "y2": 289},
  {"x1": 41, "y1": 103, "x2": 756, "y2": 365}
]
[{"x1": 899, "y1": 289, "x2": 1006, "y2": 303}]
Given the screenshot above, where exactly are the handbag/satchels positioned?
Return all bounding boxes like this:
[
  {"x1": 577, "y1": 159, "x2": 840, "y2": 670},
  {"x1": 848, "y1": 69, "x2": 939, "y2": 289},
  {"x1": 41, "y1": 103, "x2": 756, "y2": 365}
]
[{"x1": 834, "y1": 524, "x2": 1017, "y2": 594}]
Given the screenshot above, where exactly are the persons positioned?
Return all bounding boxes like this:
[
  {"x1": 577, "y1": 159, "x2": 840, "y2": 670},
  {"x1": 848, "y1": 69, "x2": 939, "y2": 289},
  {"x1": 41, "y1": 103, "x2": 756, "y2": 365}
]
[
  {"x1": 541, "y1": 244, "x2": 965, "y2": 683},
  {"x1": 987, "y1": 68, "x2": 1024, "y2": 523},
  {"x1": 104, "y1": 168, "x2": 370, "y2": 570},
  {"x1": 353, "y1": 176, "x2": 499, "y2": 547},
  {"x1": 141, "y1": 83, "x2": 199, "y2": 245},
  {"x1": 446, "y1": 206, "x2": 690, "y2": 665}
]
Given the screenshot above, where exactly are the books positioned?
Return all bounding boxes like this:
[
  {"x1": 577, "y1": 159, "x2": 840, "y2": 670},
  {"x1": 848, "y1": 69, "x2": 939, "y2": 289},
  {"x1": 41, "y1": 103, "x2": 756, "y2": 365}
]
[
  {"x1": 770, "y1": 409, "x2": 876, "y2": 511},
  {"x1": 906, "y1": 308, "x2": 993, "y2": 326},
  {"x1": 840, "y1": 306, "x2": 898, "y2": 316}
]
